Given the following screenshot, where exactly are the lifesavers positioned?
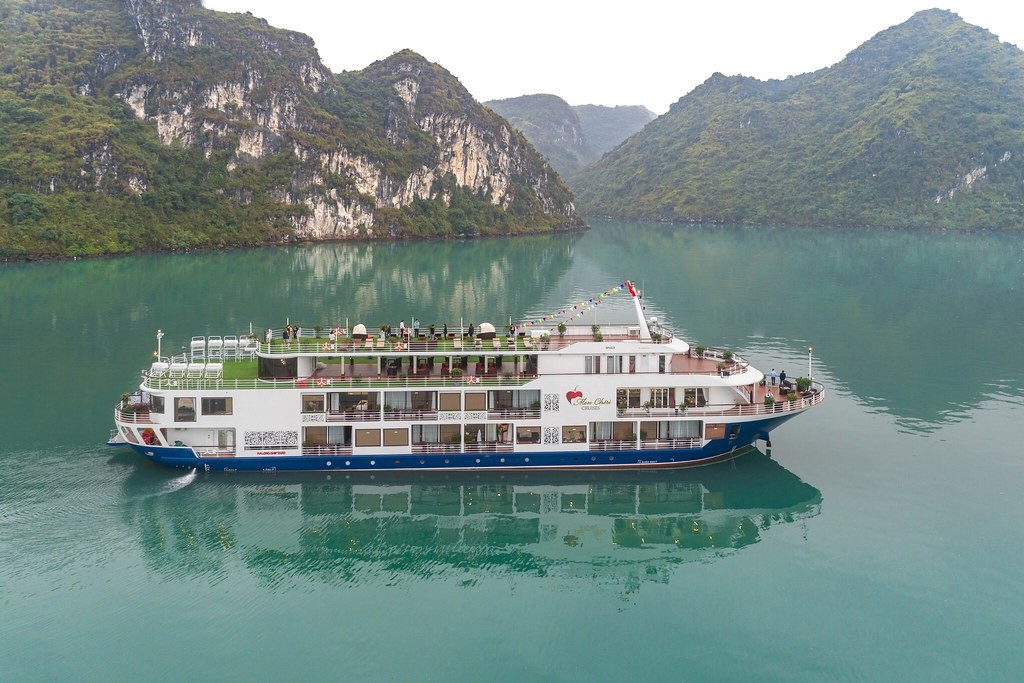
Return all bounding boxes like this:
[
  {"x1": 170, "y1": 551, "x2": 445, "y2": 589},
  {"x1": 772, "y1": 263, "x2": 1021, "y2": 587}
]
[
  {"x1": 395, "y1": 342, "x2": 404, "y2": 351},
  {"x1": 322, "y1": 343, "x2": 331, "y2": 352},
  {"x1": 334, "y1": 328, "x2": 343, "y2": 336},
  {"x1": 317, "y1": 377, "x2": 327, "y2": 387},
  {"x1": 466, "y1": 376, "x2": 476, "y2": 385},
  {"x1": 403, "y1": 327, "x2": 410, "y2": 334}
]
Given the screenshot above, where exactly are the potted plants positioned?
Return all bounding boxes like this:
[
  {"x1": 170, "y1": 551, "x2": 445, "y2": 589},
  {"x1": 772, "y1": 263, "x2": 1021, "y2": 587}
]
[
  {"x1": 558, "y1": 322, "x2": 566, "y2": 338},
  {"x1": 539, "y1": 333, "x2": 551, "y2": 350},
  {"x1": 695, "y1": 345, "x2": 706, "y2": 360},
  {"x1": 716, "y1": 351, "x2": 733, "y2": 373}
]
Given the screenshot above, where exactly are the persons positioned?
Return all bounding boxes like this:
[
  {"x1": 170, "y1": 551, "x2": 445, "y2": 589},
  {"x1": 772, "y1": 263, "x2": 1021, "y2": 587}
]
[
  {"x1": 379, "y1": 319, "x2": 474, "y2": 343},
  {"x1": 759, "y1": 374, "x2": 766, "y2": 386},
  {"x1": 769, "y1": 369, "x2": 777, "y2": 385},
  {"x1": 283, "y1": 325, "x2": 298, "y2": 350},
  {"x1": 765, "y1": 389, "x2": 774, "y2": 398},
  {"x1": 156, "y1": 403, "x2": 162, "y2": 412},
  {"x1": 618, "y1": 395, "x2": 661, "y2": 408},
  {"x1": 569, "y1": 431, "x2": 585, "y2": 440},
  {"x1": 329, "y1": 330, "x2": 335, "y2": 344},
  {"x1": 510, "y1": 324, "x2": 515, "y2": 335},
  {"x1": 779, "y1": 370, "x2": 785, "y2": 384},
  {"x1": 806, "y1": 374, "x2": 813, "y2": 391}
]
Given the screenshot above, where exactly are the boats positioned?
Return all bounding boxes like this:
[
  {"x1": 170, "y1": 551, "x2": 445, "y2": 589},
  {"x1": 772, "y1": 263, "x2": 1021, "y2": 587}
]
[
  {"x1": 103, "y1": 279, "x2": 827, "y2": 476},
  {"x1": 106, "y1": 447, "x2": 822, "y2": 591}
]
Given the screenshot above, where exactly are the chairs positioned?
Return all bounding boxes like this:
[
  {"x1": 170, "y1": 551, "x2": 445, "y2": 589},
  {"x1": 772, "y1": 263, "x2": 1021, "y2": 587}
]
[
  {"x1": 507, "y1": 338, "x2": 515, "y2": 348},
  {"x1": 404, "y1": 334, "x2": 412, "y2": 340},
  {"x1": 523, "y1": 338, "x2": 532, "y2": 347},
  {"x1": 354, "y1": 339, "x2": 361, "y2": 348},
  {"x1": 434, "y1": 333, "x2": 442, "y2": 340},
  {"x1": 392, "y1": 334, "x2": 399, "y2": 343},
  {"x1": 453, "y1": 339, "x2": 461, "y2": 348},
  {"x1": 517, "y1": 332, "x2": 526, "y2": 338},
  {"x1": 376, "y1": 338, "x2": 385, "y2": 347},
  {"x1": 340, "y1": 340, "x2": 348, "y2": 350},
  {"x1": 418, "y1": 333, "x2": 426, "y2": 340},
  {"x1": 462, "y1": 333, "x2": 468, "y2": 338},
  {"x1": 492, "y1": 338, "x2": 501, "y2": 348},
  {"x1": 149, "y1": 334, "x2": 255, "y2": 390},
  {"x1": 448, "y1": 333, "x2": 455, "y2": 340},
  {"x1": 474, "y1": 338, "x2": 483, "y2": 349},
  {"x1": 365, "y1": 339, "x2": 373, "y2": 347}
]
